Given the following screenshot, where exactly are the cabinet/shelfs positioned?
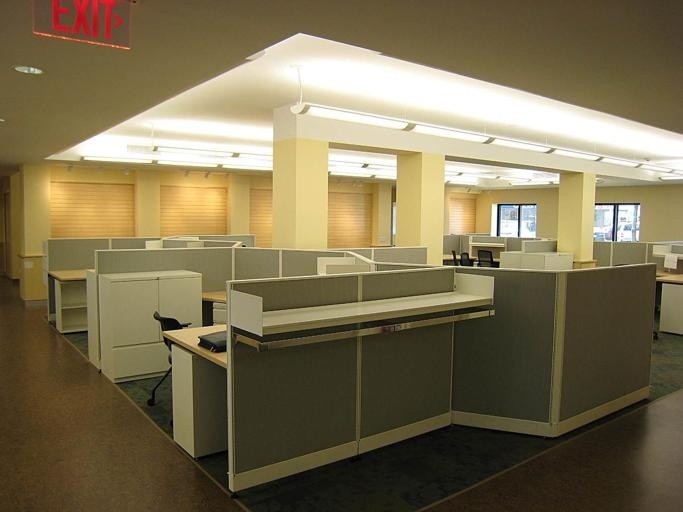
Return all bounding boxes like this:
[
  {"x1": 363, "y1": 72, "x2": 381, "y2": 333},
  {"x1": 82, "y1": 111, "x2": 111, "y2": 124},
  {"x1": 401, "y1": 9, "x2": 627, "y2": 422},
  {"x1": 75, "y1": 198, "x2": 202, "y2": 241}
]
[{"x1": 98, "y1": 270, "x2": 202, "y2": 384}]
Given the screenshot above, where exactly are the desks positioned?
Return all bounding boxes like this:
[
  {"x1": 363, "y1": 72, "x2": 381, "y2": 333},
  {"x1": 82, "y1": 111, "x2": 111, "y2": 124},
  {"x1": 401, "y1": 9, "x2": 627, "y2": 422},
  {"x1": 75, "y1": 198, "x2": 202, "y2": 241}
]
[
  {"x1": 161, "y1": 325, "x2": 227, "y2": 460},
  {"x1": 443, "y1": 254, "x2": 500, "y2": 267},
  {"x1": 656, "y1": 272, "x2": 683, "y2": 336},
  {"x1": 45, "y1": 269, "x2": 86, "y2": 333},
  {"x1": 201, "y1": 291, "x2": 229, "y2": 325}
]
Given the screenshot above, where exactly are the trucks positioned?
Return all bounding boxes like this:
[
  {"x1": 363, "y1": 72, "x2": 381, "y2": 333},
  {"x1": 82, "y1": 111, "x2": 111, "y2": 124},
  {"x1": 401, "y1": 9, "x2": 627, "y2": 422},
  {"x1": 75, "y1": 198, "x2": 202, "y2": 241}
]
[{"x1": 593, "y1": 207, "x2": 632, "y2": 241}]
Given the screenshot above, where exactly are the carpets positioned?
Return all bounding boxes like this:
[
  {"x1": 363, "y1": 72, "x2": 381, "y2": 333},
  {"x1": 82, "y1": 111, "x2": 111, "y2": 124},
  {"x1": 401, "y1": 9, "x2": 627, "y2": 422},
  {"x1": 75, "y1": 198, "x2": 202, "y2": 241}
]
[{"x1": 40, "y1": 307, "x2": 683, "y2": 512}]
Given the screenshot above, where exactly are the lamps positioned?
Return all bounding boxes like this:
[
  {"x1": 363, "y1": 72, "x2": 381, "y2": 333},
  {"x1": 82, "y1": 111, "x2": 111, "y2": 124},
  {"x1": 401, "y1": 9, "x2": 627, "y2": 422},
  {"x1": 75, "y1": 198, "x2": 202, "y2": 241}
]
[
  {"x1": 79, "y1": 137, "x2": 272, "y2": 185},
  {"x1": 290, "y1": 95, "x2": 682, "y2": 188}
]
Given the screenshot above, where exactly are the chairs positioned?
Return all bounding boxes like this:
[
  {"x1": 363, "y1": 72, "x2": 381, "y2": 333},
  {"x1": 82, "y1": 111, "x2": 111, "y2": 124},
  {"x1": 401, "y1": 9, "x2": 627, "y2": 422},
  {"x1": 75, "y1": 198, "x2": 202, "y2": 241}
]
[
  {"x1": 147, "y1": 311, "x2": 192, "y2": 406},
  {"x1": 450, "y1": 249, "x2": 495, "y2": 268}
]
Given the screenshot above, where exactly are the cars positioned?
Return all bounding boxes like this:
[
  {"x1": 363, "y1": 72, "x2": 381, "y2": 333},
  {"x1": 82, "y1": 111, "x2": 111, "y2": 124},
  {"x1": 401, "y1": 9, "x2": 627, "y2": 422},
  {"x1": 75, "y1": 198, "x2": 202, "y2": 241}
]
[{"x1": 615, "y1": 223, "x2": 639, "y2": 242}]
